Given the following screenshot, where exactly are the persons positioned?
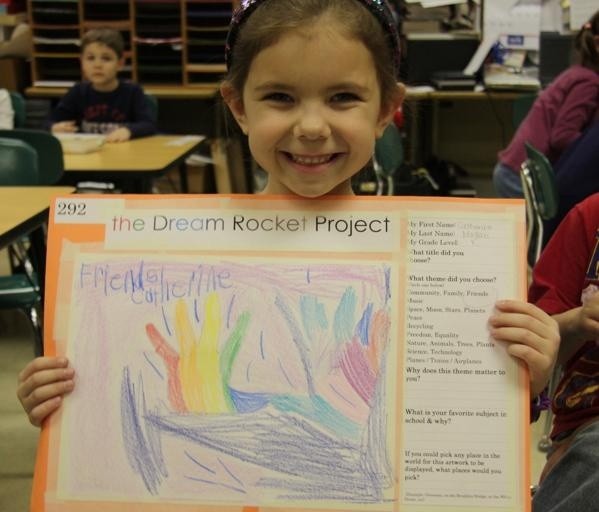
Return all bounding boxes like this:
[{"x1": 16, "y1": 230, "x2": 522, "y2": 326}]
[
  {"x1": 36, "y1": 25, "x2": 161, "y2": 196},
  {"x1": 527, "y1": 192, "x2": 599, "y2": 512},
  {"x1": 16, "y1": 1, "x2": 564, "y2": 433},
  {"x1": 489, "y1": 8, "x2": 599, "y2": 200},
  {"x1": 538, "y1": 111, "x2": 599, "y2": 254}
]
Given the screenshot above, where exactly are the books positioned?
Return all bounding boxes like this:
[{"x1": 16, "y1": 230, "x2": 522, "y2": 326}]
[{"x1": 429, "y1": 71, "x2": 545, "y2": 91}]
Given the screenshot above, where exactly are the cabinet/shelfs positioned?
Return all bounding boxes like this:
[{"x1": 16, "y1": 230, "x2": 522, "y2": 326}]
[{"x1": 27, "y1": 0, "x2": 242, "y2": 86}]
